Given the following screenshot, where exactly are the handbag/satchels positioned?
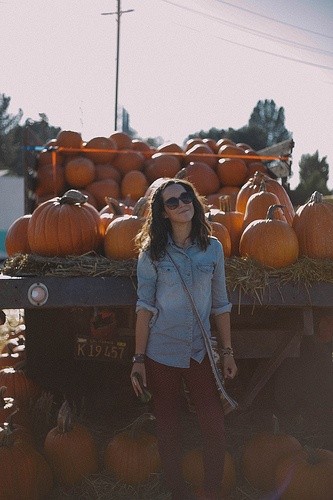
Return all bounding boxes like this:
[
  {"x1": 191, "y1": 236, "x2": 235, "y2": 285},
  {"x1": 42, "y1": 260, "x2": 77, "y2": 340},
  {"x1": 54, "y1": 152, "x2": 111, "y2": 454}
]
[{"x1": 182, "y1": 335, "x2": 239, "y2": 414}]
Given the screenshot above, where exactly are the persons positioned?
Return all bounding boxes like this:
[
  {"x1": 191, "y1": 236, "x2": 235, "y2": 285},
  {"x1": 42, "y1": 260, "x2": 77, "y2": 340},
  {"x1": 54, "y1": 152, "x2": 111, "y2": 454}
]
[{"x1": 131, "y1": 178, "x2": 237, "y2": 500}]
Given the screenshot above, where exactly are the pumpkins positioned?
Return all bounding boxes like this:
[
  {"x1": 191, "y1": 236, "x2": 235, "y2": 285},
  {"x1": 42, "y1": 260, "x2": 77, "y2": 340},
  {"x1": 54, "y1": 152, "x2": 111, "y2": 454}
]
[
  {"x1": 0, "y1": 322, "x2": 333, "y2": 500},
  {"x1": 6, "y1": 130, "x2": 333, "y2": 269}
]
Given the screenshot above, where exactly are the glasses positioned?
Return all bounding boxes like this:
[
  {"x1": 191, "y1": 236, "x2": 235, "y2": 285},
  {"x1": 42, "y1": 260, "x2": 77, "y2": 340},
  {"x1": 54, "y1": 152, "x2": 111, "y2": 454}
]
[{"x1": 164, "y1": 192, "x2": 193, "y2": 210}]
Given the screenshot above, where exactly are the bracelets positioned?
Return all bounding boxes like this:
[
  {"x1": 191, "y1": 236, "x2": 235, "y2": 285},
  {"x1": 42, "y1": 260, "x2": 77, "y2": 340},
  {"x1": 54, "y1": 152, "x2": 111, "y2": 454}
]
[
  {"x1": 132, "y1": 354, "x2": 146, "y2": 365},
  {"x1": 223, "y1": 346, "x2": 234, "y2": 358}
]
